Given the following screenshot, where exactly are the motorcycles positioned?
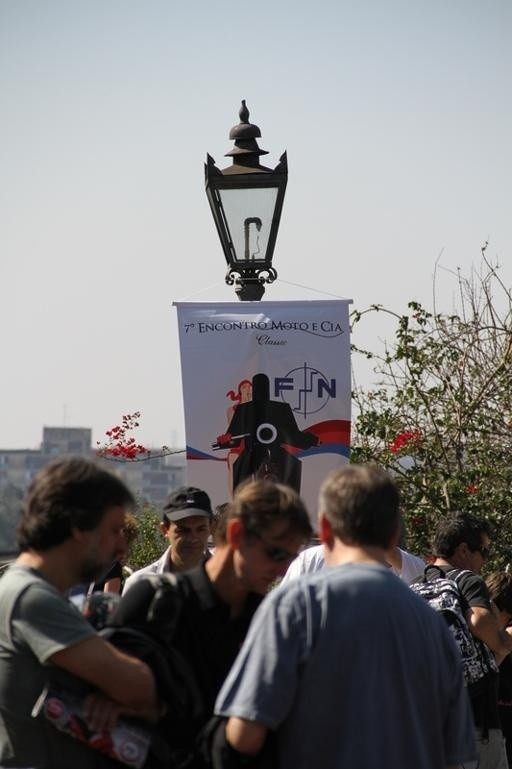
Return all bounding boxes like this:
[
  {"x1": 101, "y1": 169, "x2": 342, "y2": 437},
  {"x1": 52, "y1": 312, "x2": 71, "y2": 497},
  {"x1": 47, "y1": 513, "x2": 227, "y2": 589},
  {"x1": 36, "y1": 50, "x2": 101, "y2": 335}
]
[{"x1": 213, "y1": 427, "x2": 324, "y2": 481}]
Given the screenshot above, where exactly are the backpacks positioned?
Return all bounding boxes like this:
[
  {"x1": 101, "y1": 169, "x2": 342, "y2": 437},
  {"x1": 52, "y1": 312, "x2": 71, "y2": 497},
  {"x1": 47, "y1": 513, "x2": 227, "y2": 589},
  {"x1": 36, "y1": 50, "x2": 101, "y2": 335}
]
[{"x1": 406, "y1": 563, "x2": 500, "y2": 696}]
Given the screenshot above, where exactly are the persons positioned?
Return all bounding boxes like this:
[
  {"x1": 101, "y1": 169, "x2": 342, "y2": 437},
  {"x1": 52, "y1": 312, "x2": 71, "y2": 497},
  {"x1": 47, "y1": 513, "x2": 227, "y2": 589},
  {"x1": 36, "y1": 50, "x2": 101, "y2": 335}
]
[
  {"x1": 214, "y1": 457, "x2": 511, "y2": 769},
  {"x1": 217, "y1": 373, "x2": 320, "y2": 501},
  {"x1": 0, "y1": 456, "x2": 315, "y2": 768},
  {"x1": 214, "y1": 380, "x2": 253, "y2": 496}
]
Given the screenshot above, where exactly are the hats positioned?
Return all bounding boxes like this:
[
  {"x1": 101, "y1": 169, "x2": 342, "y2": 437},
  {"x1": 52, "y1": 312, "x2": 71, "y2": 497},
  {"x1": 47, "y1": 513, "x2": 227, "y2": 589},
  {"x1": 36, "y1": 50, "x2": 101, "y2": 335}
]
[{"x1": 162, "y1": 486, "x2": 213, "y2": 521}]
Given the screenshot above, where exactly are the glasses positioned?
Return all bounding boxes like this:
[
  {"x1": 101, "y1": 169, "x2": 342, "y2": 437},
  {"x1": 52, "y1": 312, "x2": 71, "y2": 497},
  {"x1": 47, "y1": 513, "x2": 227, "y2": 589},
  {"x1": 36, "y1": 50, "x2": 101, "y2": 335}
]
[
  {"x1": 255, "y1": 533, "x2": 299, "y2": 563},
  {"x1": 478, "y1": 544, "x2": 491, "y2": 561}
]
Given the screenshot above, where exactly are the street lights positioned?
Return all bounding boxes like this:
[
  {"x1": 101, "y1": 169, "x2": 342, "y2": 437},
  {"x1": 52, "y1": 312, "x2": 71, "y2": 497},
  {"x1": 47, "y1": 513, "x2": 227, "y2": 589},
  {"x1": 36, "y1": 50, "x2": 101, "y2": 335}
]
[{"x1": 198, "y1": 97, "x2": 290, "y2": 303}]
